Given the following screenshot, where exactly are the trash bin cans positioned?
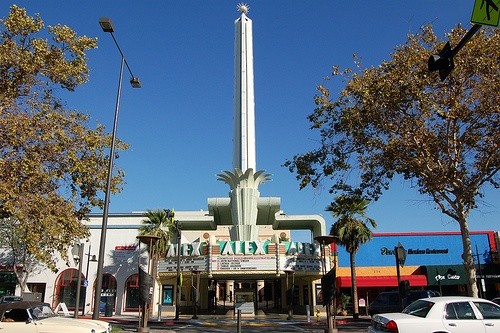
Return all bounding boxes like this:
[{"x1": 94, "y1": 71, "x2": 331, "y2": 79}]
[{"x1": 105, "y1": 303, "x2": 114, "y2": 317}]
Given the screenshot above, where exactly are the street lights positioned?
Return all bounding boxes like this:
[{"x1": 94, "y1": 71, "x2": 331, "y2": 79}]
[
  {"x1": 136, "y1": 235, "x2": 162, "y2": 333},
  {"x1": 312, "y1": 236, "x2": 338, "y2": 333},
  {"x1": 91, "y1": 17, "x2": 141, "y2": 320}
]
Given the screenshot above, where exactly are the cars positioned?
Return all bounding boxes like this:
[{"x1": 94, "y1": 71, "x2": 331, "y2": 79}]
[{"x1": 366, "y1": 295, "x2": 500, "y2": 333}]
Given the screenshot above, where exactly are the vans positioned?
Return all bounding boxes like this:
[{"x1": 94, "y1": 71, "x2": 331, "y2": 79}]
[{"x1": 368, "y1": 290, "x2": 434, "y2": 317}]
